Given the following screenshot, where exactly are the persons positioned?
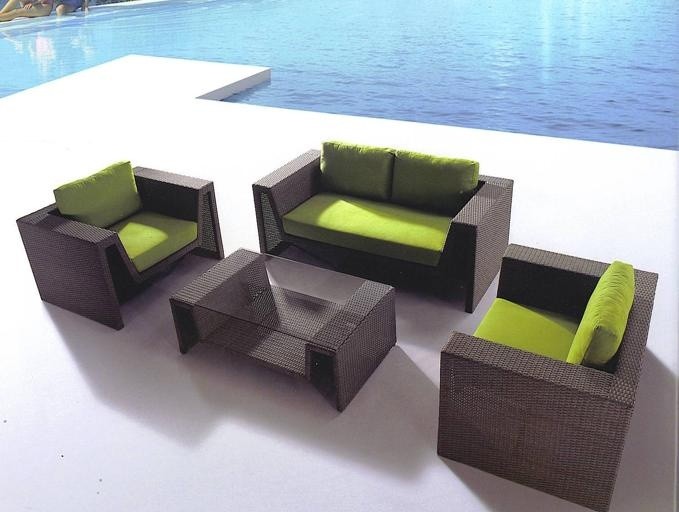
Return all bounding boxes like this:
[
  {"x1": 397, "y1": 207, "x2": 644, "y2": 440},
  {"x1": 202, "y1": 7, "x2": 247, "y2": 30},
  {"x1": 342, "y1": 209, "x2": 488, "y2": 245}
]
[
  {"x1": 51, "y1": 0, "x2": 90, "y2": 18},
  {"x1": 1, "y1": 27, "x2": 57, "y2": 79},
  {"x1": 0, "y1": 0, "x2": 54, "y2": 24},
  {"x1": 57, "y1": 18, "x2": 96, "y2": 58}
]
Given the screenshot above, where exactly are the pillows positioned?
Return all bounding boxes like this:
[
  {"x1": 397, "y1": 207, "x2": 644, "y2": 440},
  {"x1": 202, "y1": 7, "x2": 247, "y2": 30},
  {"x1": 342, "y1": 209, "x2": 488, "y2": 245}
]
[
  {"x1": 321, "y1": 141, "x2": 479, "y2": 218},
  {"x1": 560, "y1": 261, "x2": 639, "y2": 369},
  {"x1": 50, "y1": 161, "x2": 143, "y2": 229}
]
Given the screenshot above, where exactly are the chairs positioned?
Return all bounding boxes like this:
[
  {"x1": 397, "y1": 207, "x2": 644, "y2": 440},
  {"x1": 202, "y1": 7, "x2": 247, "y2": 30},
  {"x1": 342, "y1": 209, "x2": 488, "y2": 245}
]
[
  {"x1": 432, "y1": 244, "x2": 660, "y2": 511},
  {"x1": 12, "y1": 163, "x2": 224, "y2": 333}
]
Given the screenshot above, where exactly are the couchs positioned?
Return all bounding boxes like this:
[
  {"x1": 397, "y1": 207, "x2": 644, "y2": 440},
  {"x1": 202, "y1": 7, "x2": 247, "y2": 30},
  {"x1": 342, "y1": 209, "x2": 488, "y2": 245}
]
[{"x1": 252, "y1": 148, "x2": 514, "y2": 315}]
